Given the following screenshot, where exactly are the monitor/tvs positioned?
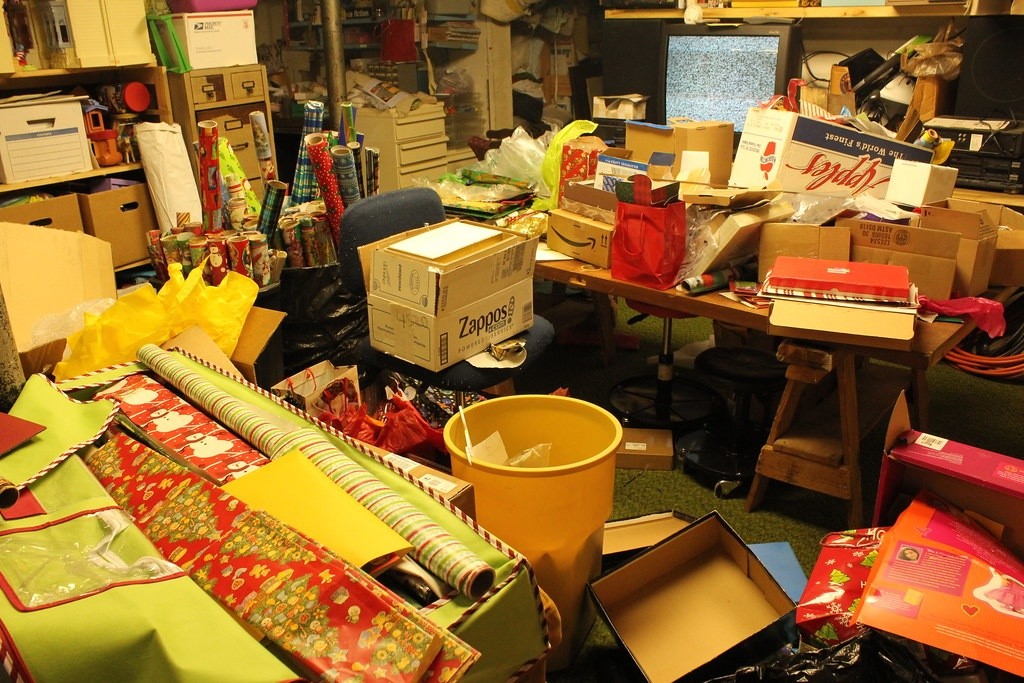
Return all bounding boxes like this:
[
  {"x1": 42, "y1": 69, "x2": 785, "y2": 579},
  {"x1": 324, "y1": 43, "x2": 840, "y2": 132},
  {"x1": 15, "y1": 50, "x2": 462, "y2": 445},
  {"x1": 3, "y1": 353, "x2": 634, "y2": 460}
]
[{"x1": 657, "y1": 21, "x2": 804, "y2": 159}]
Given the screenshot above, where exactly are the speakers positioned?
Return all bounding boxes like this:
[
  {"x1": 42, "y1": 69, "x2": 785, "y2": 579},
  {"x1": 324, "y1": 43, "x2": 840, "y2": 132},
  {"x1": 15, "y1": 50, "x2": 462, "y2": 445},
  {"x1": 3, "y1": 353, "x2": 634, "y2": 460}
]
[{"x1": 955, "y1": 13, "x2": 1024, "y2": 121}]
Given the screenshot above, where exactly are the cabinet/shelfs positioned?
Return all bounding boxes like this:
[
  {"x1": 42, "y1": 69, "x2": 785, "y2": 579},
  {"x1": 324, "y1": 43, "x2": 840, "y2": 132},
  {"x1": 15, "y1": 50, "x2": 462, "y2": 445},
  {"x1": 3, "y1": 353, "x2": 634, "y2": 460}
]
[
  {"x1": 167, "y1": 66, "x2": 279, "y2": 202},
  {"x1": 349, "y1": 102, "x2": 448, "y2": 191}
]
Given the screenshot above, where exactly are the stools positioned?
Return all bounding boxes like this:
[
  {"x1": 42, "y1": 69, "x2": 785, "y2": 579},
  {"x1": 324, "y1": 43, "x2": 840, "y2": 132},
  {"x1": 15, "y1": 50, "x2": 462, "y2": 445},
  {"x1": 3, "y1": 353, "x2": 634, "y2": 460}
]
[{"x1": 613, "y1": 294, "x2": 715, "y2": 428}]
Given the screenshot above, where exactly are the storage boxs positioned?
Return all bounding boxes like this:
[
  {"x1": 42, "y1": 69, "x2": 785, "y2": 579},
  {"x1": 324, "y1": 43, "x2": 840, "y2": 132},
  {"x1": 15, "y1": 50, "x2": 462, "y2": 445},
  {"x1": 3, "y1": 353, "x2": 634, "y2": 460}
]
[
  {"x1": 593, "y1": 96, "x2": 646, "y2": 120},
  {"x1": 587, "y1": 510, "x2": 797, "y2": 683},
  {"x1": 160, "y1": 10, "x2": 258, "y2": 68},
  {"x1": 358, "y1": 218, "x2": 539, "y2": 372},
  {"x1": 548, "y1": 107, "x2": 1024, "y2": 555},
  {"x1": 0, "y1": 95, "x2": 160, "y2": 274}
]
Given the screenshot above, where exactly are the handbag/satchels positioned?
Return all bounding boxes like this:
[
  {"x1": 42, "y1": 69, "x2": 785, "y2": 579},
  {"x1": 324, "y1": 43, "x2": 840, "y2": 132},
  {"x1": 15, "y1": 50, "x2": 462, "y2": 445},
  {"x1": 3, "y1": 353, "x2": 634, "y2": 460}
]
[
  {"x1": 557, "y1": 135, "x2": 608, "y2": 212},
  {"x1": 270, "y1": 359, "x2": 360, "y2": 420},
  {"x1": 610, "y1": 199, "x2": 687, "y2": 290},
  {"x1": 372, "y1": 0, "x2": 416, "y2": 61}
]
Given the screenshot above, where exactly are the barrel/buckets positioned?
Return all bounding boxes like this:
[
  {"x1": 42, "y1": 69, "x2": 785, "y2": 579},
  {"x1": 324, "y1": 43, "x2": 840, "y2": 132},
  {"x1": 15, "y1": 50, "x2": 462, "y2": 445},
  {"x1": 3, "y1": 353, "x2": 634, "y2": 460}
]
[{"x1": 442, "y1": 394, "x2": 622, "y2": 672}]
